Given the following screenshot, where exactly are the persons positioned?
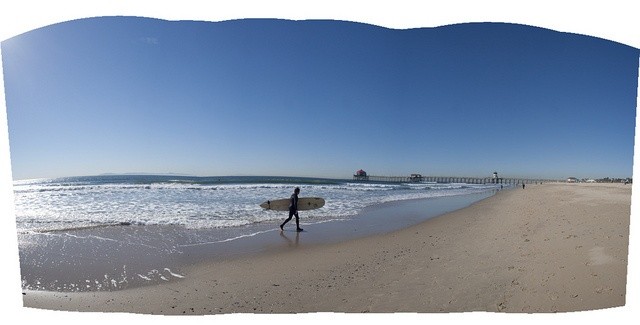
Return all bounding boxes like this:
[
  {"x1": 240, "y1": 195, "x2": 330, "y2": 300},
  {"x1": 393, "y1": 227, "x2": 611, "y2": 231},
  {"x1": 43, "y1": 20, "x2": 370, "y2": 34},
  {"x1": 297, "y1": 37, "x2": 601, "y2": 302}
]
[{"x1": 280, "y1": 187, "x2": 303, "y2": 232}]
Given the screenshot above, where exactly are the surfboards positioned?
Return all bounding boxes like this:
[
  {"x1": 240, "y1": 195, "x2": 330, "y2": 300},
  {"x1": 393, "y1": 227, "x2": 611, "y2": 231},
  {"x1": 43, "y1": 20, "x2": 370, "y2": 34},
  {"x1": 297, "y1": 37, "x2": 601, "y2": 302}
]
[{"x1": 260, "y1": 198, "x2": 325, "y2": 211}]
